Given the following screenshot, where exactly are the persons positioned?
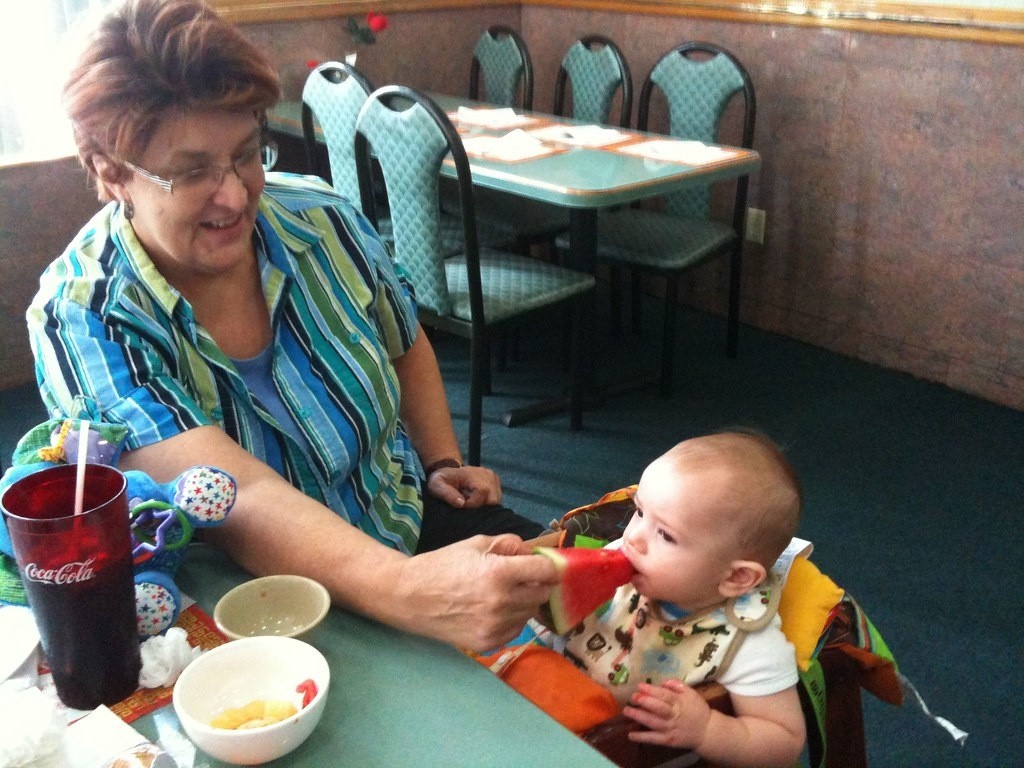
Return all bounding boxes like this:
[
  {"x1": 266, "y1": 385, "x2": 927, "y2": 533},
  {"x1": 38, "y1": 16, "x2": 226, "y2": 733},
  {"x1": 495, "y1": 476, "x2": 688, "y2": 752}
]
[
  {"x1": 28, "y1": 0, "x2": 564, "y2": 655},
  {"x1": 533, "y1": 431, "x2": 806, "y2": 768}
]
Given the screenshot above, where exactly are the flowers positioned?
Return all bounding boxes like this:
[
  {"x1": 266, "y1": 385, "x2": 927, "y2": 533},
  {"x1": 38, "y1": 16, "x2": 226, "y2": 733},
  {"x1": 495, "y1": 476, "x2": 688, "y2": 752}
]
[{"x1": 344, "y1": 9, "x2": 391, "y2": 68}]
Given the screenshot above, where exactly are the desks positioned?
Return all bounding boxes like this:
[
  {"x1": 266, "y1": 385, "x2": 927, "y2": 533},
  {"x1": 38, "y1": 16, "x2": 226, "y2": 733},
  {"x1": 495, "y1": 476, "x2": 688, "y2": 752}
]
[{"x1": 263, "y1": 88, "x2": 761, "y2": 431}]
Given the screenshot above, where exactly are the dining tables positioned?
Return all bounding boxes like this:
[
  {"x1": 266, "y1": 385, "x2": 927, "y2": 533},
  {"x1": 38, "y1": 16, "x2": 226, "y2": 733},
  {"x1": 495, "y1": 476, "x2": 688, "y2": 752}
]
[{"x1": 0, "y1": 538, "x2": 623, "y2": 768}]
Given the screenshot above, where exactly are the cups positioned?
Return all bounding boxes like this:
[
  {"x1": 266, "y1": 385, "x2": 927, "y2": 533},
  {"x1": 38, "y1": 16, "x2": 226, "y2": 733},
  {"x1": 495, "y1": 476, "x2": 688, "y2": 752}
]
[{"x1": 0, "y1": 465, "x2": 141, "y2": 712}]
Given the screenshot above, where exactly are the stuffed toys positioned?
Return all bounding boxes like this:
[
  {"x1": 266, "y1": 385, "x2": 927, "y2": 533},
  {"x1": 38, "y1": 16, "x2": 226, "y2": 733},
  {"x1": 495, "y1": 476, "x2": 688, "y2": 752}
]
[{"x1": 0, "y1": 419, "x2": 238, "y2": 642}]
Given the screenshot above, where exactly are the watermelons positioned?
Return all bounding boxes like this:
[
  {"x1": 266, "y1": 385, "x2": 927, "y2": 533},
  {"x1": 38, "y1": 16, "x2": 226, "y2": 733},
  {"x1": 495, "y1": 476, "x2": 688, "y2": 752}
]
[{"x1": 531, "y1": 547, "x2": 638, "y2": 635}]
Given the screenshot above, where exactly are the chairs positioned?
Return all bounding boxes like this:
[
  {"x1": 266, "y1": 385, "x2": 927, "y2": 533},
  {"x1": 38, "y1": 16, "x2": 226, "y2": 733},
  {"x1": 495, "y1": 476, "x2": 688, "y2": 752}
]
[{"x1": 298, "y1": 22, "x2": 755, "y2": 468}]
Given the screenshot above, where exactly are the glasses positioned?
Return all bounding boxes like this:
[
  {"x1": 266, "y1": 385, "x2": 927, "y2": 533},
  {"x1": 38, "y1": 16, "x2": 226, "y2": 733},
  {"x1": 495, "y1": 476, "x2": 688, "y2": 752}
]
[{"x1": 101, "y1": 134, "x2": 279, "y2": 202}]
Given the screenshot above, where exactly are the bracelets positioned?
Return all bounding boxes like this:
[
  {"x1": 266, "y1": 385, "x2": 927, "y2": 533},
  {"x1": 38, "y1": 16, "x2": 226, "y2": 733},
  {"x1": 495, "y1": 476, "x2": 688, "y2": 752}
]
[{"x1": 425, "y1": 458, "x2": 466, "y2": 477}]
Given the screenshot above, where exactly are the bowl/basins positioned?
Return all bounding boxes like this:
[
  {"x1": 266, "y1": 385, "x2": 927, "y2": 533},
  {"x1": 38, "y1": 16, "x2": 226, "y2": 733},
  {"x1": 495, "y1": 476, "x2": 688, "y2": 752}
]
[
  {"x1": 212, "y1": 575, "x2": 331, "y2": 637},
  {"x1": 172, "y1": 636, "x2": 330, "y2": 764}
]
